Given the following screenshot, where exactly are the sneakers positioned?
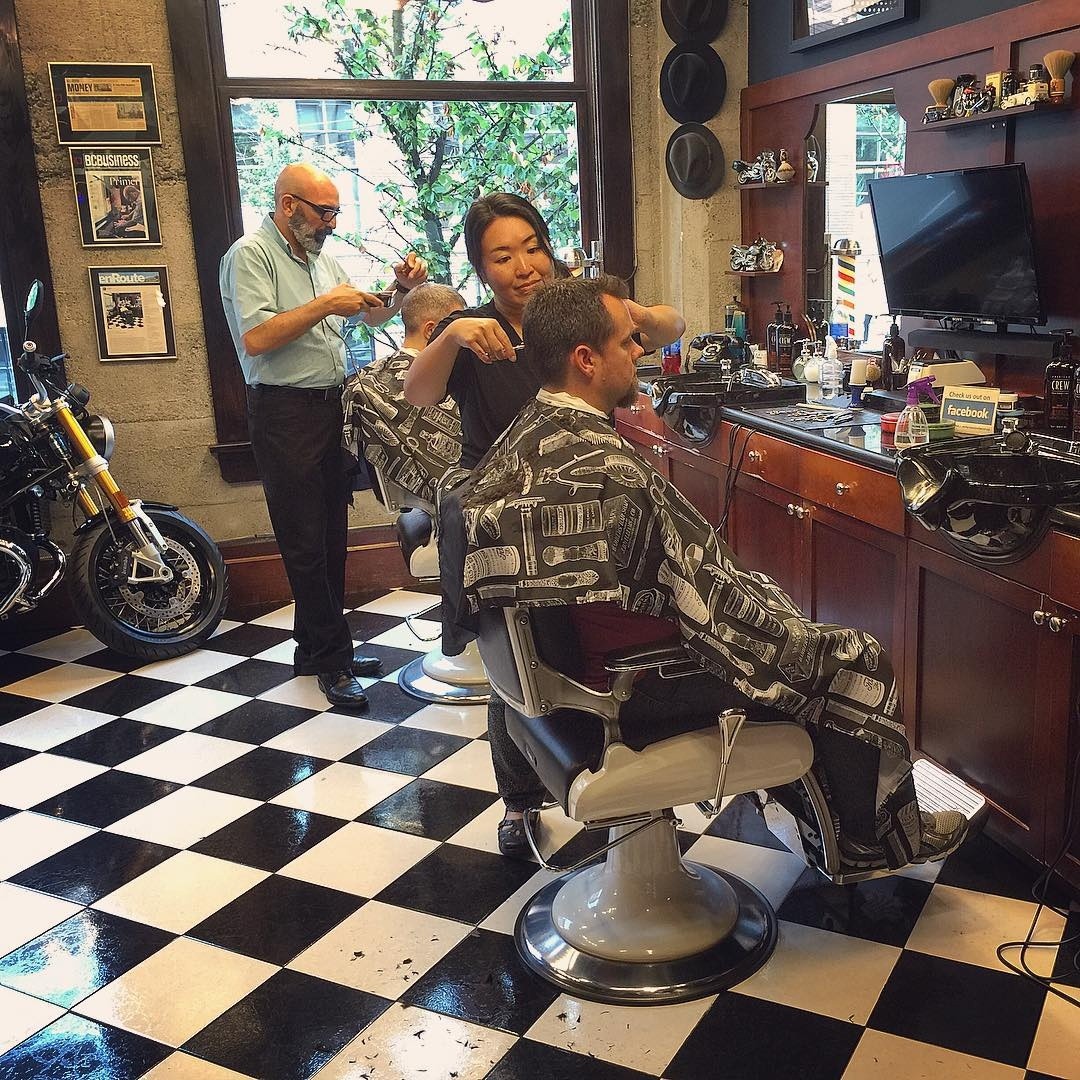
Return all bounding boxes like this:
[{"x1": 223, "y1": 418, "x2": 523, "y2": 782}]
[{"x1": 839, "y1": 811, "x2": 970, "y2": 869}]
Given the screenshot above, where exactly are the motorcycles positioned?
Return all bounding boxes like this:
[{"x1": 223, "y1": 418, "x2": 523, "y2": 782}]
[{"x1": 1, "y1": 277, "x2": 231, "y2": 657}]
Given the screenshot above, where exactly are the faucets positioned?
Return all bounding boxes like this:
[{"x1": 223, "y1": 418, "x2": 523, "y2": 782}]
[
  {"x1": 743, "y1": 367, "x2": 781, "y2": 388},
  {"x1": 1000, "y1": 417, "x2": 1019, "y2": 452},
  {"x1": 720, "y1": 359, "x2": 731, "y2": 379}
]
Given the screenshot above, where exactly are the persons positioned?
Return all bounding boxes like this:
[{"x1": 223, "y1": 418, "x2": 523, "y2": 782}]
[
  {"x1": 218, "y1": 162, "x2": 429, "y2": 709},
  {"x1": 402, "y1": 193, "x2": 686, "y2": 859},
  {"x1": 113, "y1": 186, "x2": 144, "y2": 229},
  {"x1": 457, "y1": 274, "x2": 971, "y2": 869},
  {"x1": 398, "y1": 283, "x2": 468, "y2": 359}
]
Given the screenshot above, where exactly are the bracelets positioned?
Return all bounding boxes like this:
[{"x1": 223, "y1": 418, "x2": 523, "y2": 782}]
[{"x1": 392, "y1": 279, "x2": 411, "y2": 294}]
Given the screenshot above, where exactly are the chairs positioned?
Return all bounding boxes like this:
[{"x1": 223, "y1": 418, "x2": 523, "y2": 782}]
[
  {"x1": 344, "y1": 362, "x2": 490, "y2": 705},
  {"x1": 438, "y1": 493, "x2": 990, "y2": 1007}
]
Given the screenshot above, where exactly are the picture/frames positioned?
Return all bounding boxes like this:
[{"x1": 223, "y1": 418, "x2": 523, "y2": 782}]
[
  {"x1": 47, "y1": 62, "x2": 162, "y2": 144},
  {"x1": 785, "y1": 0, "x2": 920, "y2": 56},
  {"x1": 88, "y1": 265, "x2": 178, "y2": 362},
  {"x1": 68, "y1": 147, "x2": 163, "y2": 247}
]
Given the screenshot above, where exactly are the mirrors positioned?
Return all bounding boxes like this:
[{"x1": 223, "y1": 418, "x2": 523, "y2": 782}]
[{"x1": 802, "y1": 87, "x2": 906, "y2": 356}]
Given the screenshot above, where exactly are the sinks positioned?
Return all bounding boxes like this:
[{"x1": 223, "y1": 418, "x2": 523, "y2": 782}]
[
  {"x1": 660, "y1": 372, "x2": 759, "y2": 408},
  {"x1": 895, "y1": 428, "x2": 1079, "y2": 505}
]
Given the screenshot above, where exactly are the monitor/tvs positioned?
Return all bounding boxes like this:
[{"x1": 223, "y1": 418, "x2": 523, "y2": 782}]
[{"x1": 865, "y1": 161, "x2": 1047, "y2": 333}]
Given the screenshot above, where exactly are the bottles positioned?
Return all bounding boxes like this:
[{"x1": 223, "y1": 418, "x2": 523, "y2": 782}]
[
  {"x1": 662, "y1": 338, "x2": 681, "y2": 371},
  {"x1": 829, "y1": 299, "x2": 849, "y2": 351}
]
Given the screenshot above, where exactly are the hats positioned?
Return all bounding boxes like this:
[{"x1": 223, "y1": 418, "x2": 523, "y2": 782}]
[
  {"x1": 660, "y1": 0, "x2": 729, "y2": 51},
  {"x1": 665, "y1": 122, "x2": 725, "y2": 199},
  {"x1": 660, "y1": 42, "x2": 727, "y2": 126}
]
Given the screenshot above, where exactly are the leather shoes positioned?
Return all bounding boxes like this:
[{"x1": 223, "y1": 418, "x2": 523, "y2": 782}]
[
  {"x1": 316, "y1": 670, "x2": 369, "y2": 707},
  {"x1": 294, "y1": 652, "x2": 384, "y2": 675}
]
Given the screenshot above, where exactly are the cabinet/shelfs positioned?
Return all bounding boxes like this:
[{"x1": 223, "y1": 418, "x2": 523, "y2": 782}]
[
  {"x1": 615, "y1": 394, "x2": 1080, "y2": 891},
  {"x1": 908, "y1": 104, "x2": 1064, "y2": 358},
  {"x1": 724, "y1": 184, "x2": 792, "y2": 278}
]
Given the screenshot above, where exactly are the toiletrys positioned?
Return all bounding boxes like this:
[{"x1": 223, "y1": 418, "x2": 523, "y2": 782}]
[
  {"x1": 830, "y1": 299, "x2": 849, "y2": 341},
  {"x1": 807, "y1": 297, "x2": 832, "y2": 344},
  {"x1": 893, "y1": 374, "x2": 940, "y2": 450},
  {"x1": 792, "y1": 337, "x2": 825, "y2": 383},
  {"x1": 879, "y1": 312, "x2": 905, "y2": 391},
  {"x1": 822, "y1": 336, "x2": 844, "y2": 399},
  {"x1": 1041, "y1": 329, "x2": 1080, "y2": 440},
  {"x1": 765, "y1": 301, "x2": 800, "y2": 371}
]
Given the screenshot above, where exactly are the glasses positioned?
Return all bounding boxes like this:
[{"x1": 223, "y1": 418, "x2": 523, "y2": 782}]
[{"x1": 283, "y1": 192, "x2": 341, "y2": 223}]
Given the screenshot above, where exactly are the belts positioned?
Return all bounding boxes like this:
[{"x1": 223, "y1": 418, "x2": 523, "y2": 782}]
[{"x1": 248, "y1": 381, "x2": 344, "y2": 401}]
